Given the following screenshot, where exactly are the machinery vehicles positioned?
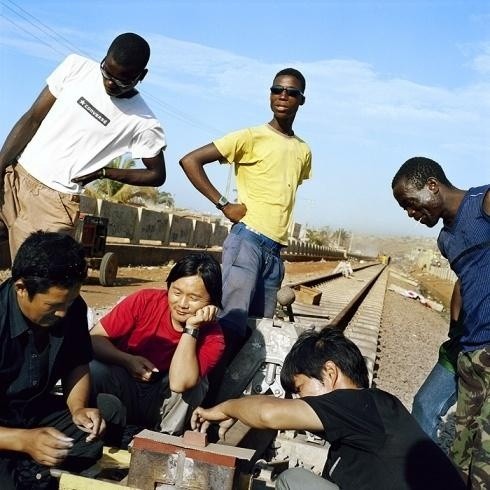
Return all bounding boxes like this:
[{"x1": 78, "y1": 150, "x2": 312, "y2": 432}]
[{"x1": 74, "y1": 213, "x2": 117, "y2": 286}]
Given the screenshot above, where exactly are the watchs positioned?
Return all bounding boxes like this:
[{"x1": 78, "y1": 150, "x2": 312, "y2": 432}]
[{"x1": 216, "y1": 196, "x2": 228, "y2": 210}]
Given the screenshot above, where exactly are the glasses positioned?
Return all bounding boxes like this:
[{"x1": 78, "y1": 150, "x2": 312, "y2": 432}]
[
  {"x1": 271, "y1": 86, "x2": 304, "y2": 99},
  {"x1": 99, "y1": 56, "x2": 142, "y2": 89}
]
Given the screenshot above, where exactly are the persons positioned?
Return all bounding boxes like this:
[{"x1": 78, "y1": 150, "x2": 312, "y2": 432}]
[
  {"x1": 391, "y1": 156, "x2": 490, "y2": 490},
  {"x1": 179, "y1": 69, "x2": 312, "y2": 323},
  {"x1": 0, "y1": 32, "x2": 167, "y2": 269},
  {"x1": 411, "y1": 279, "x2": 463, "y2": 449},
  {"x1": 89, "y1": 251, "x2": 226, "y2": 448},
  {"x1": 0, "y1": 229, "x2": 106, "y2": 490},
  {"x1": 190, "y1": 326, "x2": 470, "y2": 490}
]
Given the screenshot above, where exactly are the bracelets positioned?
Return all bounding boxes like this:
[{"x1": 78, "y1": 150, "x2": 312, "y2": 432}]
[
  {"x1": 102, "y1": 167, "x2": 106, "y2": 177},
  {"x1": 182, "y1": 328, "x2": 200, "y2": 339}
]
